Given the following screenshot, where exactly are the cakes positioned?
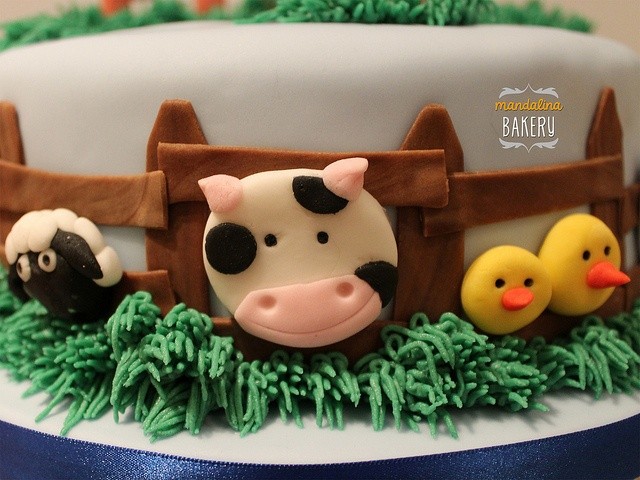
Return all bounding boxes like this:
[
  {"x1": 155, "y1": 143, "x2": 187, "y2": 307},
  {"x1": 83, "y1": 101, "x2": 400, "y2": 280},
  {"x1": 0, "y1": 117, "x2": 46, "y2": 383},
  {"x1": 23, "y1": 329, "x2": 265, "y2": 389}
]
[{"x1": 1, "y1": 0, "x2": 639, "y2": 463}]
